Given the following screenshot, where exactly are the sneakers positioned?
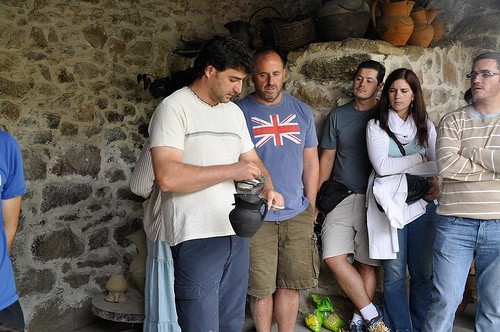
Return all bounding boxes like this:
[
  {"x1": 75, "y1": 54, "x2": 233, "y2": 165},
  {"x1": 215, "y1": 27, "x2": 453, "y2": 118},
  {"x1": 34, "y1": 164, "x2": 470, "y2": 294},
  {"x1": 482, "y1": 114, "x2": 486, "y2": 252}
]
[
  {"x1": 350, "y1": 320, "x2": 366, "y2": 332},
  {"x1": 364, "y1": 304, "x2": 390, "y2": 332}
]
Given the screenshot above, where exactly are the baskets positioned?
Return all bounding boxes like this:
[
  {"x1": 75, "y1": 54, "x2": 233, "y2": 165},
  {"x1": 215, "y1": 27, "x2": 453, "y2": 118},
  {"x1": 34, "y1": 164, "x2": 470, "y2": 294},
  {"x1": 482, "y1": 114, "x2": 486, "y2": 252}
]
[{"x1": 269, "y1": 15, "x2": 314, "y2": 53}]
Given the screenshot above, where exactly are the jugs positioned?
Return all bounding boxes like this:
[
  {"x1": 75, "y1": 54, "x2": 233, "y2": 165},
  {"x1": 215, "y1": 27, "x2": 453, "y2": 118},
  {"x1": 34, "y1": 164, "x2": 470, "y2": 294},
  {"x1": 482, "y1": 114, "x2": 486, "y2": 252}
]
[
  {"x1": 378, "y1": 0, "x2": 445, "y2": 48},
  {"x1": 229, "y1": 193, "x2": 268, "y2": 238}
]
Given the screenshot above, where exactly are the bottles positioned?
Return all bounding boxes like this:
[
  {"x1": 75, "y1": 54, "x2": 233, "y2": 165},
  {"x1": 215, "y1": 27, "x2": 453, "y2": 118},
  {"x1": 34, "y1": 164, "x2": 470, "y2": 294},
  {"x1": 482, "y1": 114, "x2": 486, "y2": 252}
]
[
  {"x1": 149, "y1": 78, "x2": 173, "y2": 99},
  {"x1": 224, "y1": 20, "x2": 252, "y2": 45},
  {"x1": 316, "y1": 0, "x2": 372, "y2": 40}
]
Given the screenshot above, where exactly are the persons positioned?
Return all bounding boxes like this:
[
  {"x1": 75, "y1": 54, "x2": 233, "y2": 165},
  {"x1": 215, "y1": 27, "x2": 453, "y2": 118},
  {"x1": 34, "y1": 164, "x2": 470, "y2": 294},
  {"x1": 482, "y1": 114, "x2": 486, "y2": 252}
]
[
  {"x1": 314, "y1": 61, "x2": 398, "y2": 332},
  {"x1": 422, "y1": 51, "x2": 500, "y2": 332},
  {"x1": 365, "y1": 69, "x2": 438, "y2": 332},
  {"x1": 0, "y1": 130, "x2": 26, "y2": 332},
  {"x1": 147, "y1": 37, "x2": 284, "y2": 332},
  {"x1": 237, "y1": 48, "x2": 320, "y2": 332}
]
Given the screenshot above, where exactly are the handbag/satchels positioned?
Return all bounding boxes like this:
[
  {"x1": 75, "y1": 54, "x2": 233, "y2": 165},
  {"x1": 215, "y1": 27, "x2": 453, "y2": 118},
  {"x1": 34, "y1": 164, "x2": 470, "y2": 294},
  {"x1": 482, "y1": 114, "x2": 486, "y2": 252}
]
[
  {"x1": 372, "y1": 119, "x2": 430, "y2": 212},
  {"x1": 316, "y1": 181, "x2": 353, "y2": 213}
]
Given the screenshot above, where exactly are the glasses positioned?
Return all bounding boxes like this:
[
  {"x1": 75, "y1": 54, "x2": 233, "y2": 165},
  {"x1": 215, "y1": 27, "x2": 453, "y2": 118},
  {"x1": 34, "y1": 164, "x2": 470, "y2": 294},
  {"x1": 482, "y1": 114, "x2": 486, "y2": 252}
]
[{"x1": 466, "y1": 71, "x2": 500, "y2": 78}]
